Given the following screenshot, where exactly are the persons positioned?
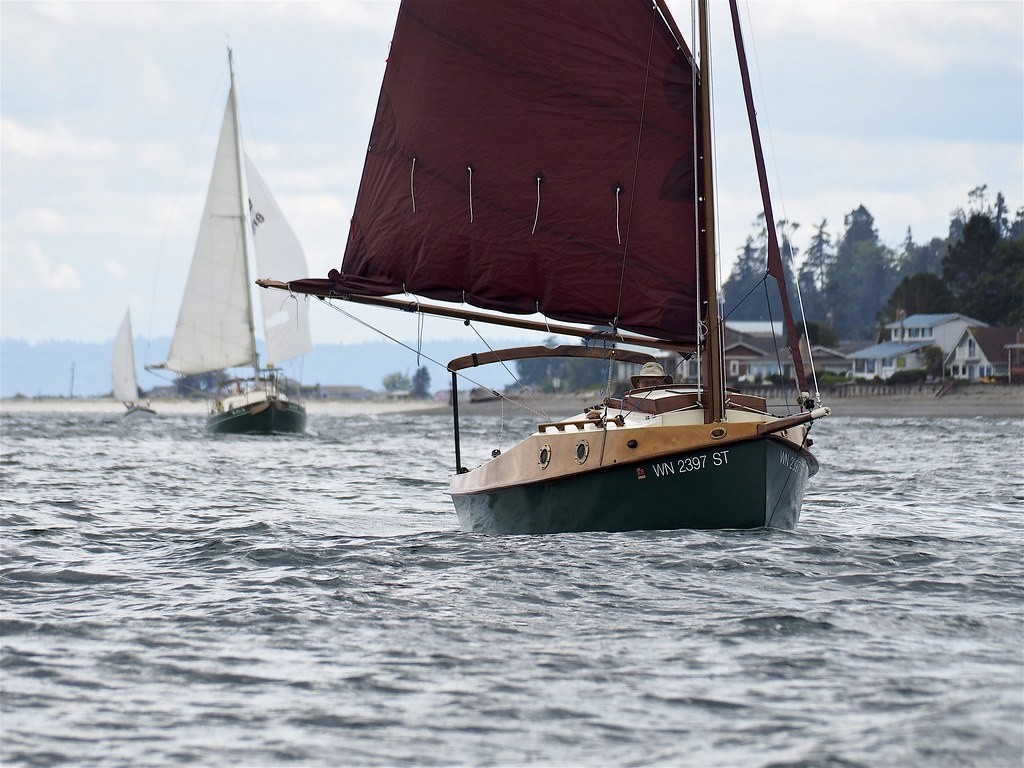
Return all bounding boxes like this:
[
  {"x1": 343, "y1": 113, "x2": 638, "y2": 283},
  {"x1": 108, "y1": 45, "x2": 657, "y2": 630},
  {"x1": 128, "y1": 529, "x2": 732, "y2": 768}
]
[{"x1": 630, "y1": 362, "x2": 673, "y2": 388}]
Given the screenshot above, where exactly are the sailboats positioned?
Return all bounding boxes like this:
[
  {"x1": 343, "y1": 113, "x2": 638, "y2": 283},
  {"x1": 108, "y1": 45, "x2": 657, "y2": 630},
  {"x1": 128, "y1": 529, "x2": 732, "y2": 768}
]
[
  {"x1": 131, "y1": 45, "x2": 311, "y2": 436},
  {"x1": 110, "y1": 305, "x2": 160, "y2": 421},
  {"x1": 255, "y1": 0, "x2": 828, "y2": 531}
]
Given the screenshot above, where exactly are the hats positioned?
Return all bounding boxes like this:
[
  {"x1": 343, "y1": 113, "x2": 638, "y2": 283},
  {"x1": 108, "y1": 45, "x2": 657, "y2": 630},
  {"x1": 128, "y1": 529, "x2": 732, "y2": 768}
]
[{"x1": 630, "y1": 362, "x2": 674, "y2": 388}]
